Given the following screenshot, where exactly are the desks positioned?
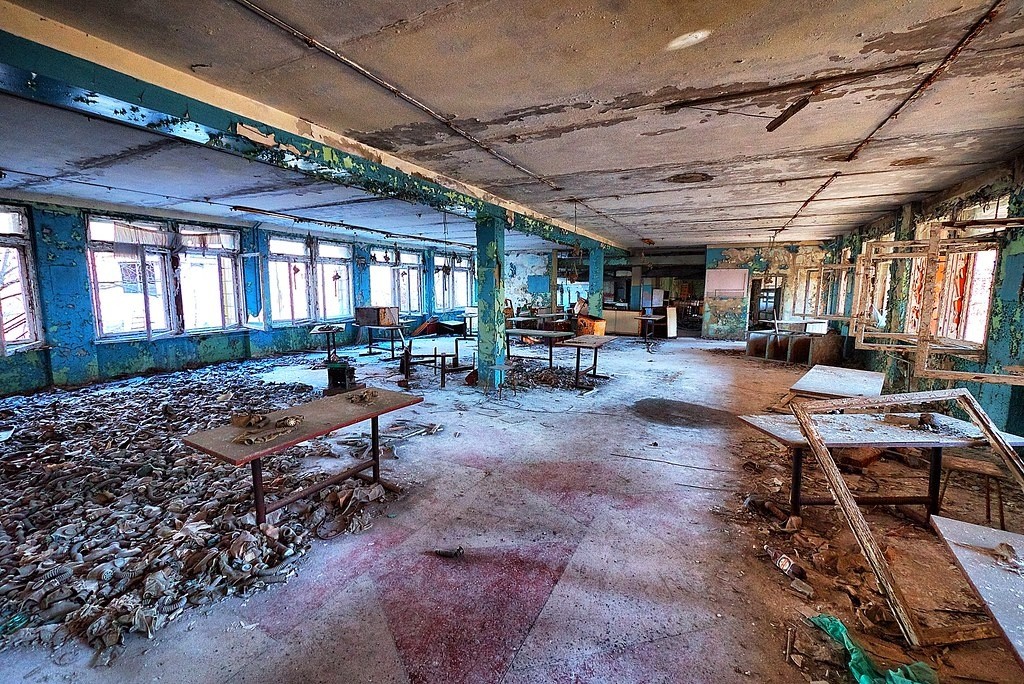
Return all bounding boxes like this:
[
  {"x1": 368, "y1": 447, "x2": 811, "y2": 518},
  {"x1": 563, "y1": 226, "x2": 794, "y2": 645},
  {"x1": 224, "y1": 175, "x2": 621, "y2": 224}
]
[
  {"x1": 735, "y1": 411, "x2": 1024, "y2": 531},
  {"x1": 311, "y1": 313, "x2": 666, "y2": 390},
  {"x1": 182, "y1": 386, "x2": 423, "y2": 528},
  {"x1": 788, "y1": 364, "x2": 887, "y2": 475}
]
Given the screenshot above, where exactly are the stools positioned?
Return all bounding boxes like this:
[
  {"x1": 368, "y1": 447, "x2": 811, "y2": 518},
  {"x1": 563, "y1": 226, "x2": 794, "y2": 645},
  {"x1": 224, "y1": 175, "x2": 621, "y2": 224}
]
[{"x1": 943, "y1": 454, "x2": 1008, "y2": 531}]
